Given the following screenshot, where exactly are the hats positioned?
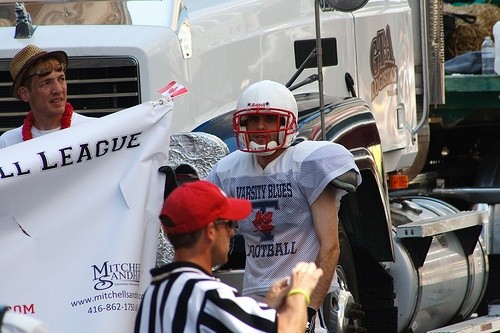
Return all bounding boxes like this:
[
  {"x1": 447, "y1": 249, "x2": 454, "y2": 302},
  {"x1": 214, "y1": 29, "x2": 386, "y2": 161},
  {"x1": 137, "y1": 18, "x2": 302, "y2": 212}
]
[
  {"x1": 8, "y1": 45, "x2": 69, "y2": 100},
  {"x1": 158, "y1": 180, "x2": 252, "y2": 235}
]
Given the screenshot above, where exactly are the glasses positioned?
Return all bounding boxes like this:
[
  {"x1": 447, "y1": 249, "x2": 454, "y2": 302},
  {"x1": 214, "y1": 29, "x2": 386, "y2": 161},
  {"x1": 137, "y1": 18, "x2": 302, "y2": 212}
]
[
  {"x1": 213, "y1": 219, "x2": 238, "y2": 230},
  {"x1": 21, "y1": 63, "x2": 64, "y2": 83}
]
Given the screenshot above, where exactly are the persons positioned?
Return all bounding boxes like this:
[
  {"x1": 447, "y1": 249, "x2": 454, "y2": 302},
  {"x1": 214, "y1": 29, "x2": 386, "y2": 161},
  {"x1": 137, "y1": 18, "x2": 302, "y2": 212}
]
[
  {"x1": 0, "y1": 44, "x2": 90, "y2": 150},
  {"x1": 157, "y1": 80, "x2": 362, "y2": 333},
  {"x1": 133, "y1": 183, "x2": 323, "y2": 333}
]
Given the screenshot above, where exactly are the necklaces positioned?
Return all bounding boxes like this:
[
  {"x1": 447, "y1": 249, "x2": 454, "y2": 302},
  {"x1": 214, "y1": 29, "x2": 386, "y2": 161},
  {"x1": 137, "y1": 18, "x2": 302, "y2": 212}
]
[{"x1": 23, "y1": 103, "x2": 72, "y2": 142}]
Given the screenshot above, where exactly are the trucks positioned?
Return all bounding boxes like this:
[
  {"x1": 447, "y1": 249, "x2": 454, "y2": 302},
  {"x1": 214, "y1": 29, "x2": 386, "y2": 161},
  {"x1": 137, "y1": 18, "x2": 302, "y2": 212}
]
[{"x1": 0, "y1": 0, "x2": 500, "y2": 333}]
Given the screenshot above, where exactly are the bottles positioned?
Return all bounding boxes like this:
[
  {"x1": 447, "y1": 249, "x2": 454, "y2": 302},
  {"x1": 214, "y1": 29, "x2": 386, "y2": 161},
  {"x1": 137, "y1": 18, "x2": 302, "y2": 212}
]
[
  {"x1": 492, "y1": 20, "x2": 500, "y2": 76},
  {"x1": 481, "y1": 36, "x2": 495, "y2": 75}
]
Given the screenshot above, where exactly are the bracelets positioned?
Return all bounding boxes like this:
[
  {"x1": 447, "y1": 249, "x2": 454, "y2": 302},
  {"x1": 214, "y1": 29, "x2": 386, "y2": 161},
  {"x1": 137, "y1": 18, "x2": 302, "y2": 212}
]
[{"x1": 286, "y1": 289, "x2": 311, "y2": 306}]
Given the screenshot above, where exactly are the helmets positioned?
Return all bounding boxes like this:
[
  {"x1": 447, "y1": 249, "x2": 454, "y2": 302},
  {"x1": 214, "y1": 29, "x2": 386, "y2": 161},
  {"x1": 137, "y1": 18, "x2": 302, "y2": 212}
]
[{"x1": 233, "y1": 80, "x2": 299, "y2": 156}]
